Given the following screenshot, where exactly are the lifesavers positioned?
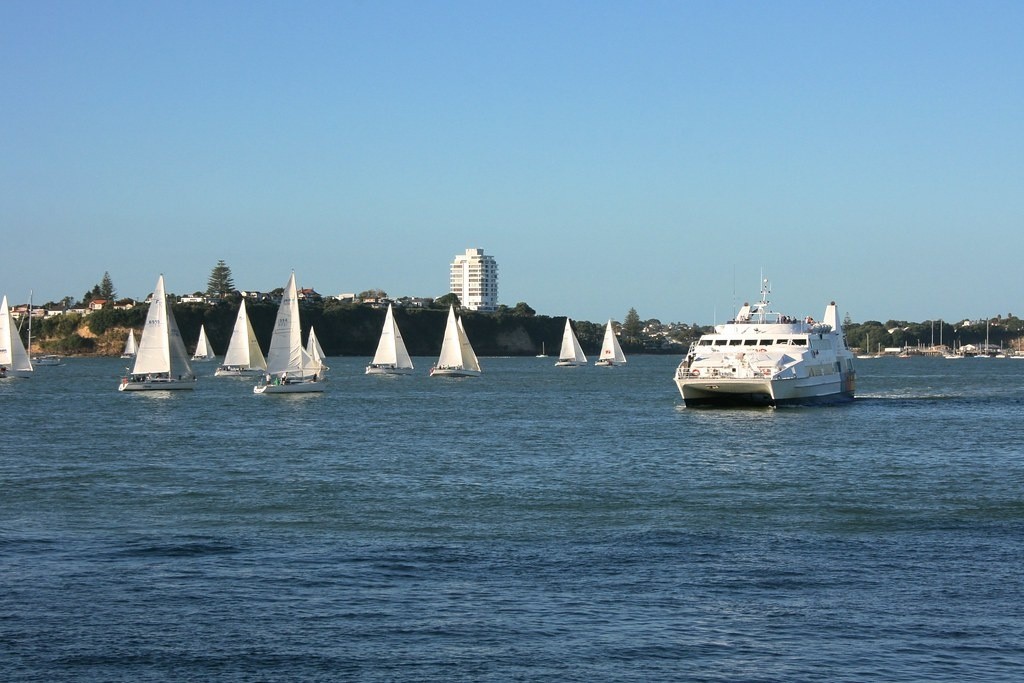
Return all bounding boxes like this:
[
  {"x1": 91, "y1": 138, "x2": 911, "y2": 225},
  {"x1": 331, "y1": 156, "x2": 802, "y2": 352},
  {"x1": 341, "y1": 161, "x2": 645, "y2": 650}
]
[{"x1": 692, "y1": 369, "x2": 701, "y2": 377}]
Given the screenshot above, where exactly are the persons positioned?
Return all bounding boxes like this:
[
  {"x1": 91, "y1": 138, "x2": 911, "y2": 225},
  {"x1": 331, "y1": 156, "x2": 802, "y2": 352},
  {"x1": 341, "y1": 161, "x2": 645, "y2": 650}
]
[
  {"x1": 0, "y1": 366, "x2": 6, "y2": 373},
  {"x1": 783, "y1": 315, "x2": 796, "y2": 324},
  {"x1": 266, "y1": 373, "x2": 271, "y2": 384},
  {"x1": 439, "y1": 364, "x2": 449, "y2": 369},
  {"x1": 282, "y1": 372, "x2": 288, "y2": 386},
  {"x1": 223, "y1": 365, "x2": 231, "y2": 371},
  {"x1": 137, "y1": 373, "x2": 162, "y2": 382},
  {"x1": 805, "y1": 316, "x2": 811, "y2": 324}
]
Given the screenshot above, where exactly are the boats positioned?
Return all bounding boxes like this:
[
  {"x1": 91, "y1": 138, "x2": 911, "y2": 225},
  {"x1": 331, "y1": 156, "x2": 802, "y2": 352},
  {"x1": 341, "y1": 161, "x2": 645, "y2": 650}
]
[{"x1": 670, "y1": 268, "x2": 857, "y2": 411}]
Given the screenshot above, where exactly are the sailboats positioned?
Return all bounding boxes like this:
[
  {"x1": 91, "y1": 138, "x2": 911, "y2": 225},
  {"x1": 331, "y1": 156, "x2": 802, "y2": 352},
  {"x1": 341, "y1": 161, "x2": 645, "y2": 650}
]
[
  {"x1": 430, "y1": 302, "x2": 483, "y2": 378},
  {"x1": 253, "y1": 267, "x2": 332, "y2": 394},
  {"x1": 898, "y1": 341, "x2": 912, "y2": 358},
  {"x1": 189, "y1": 323, "x2": 218, "y2": 363},
  {"x1": 873, "y1": 342, "x2": 882, "y2": 359},
  {"x1": 118, "y1": 273, "x2": 200, "y2": 392},
  {"x1": 535, "y1": 341, "x2": 549, "y2": 358},
  {"x1": 363, "y1": 302, "x2": 416, "y2": 376},
  {"x1": 942, "y1": 319, "x2": 1024, "y2": 360},
  {"x1": 15, "y1": 290, "x2": 63, "y2": 367},
  {"x1": 0, "y1": 294, "x2": 35, "y2": 380},
  {"x1": 856, "y1": 331, "x2": 875, "y2": 359},
  {"x1": 593, "y1": 318, "x2": 628, "y2": 368},
  {"x1": 120, "y1": 327, "x2": 139, "y2": 360},
  {"x1": 212, "y1": 298, "x2": 268, "y2": 378},
  {"x1": 554, "y1": 317, "x2": 588, "y2": 366}
]
